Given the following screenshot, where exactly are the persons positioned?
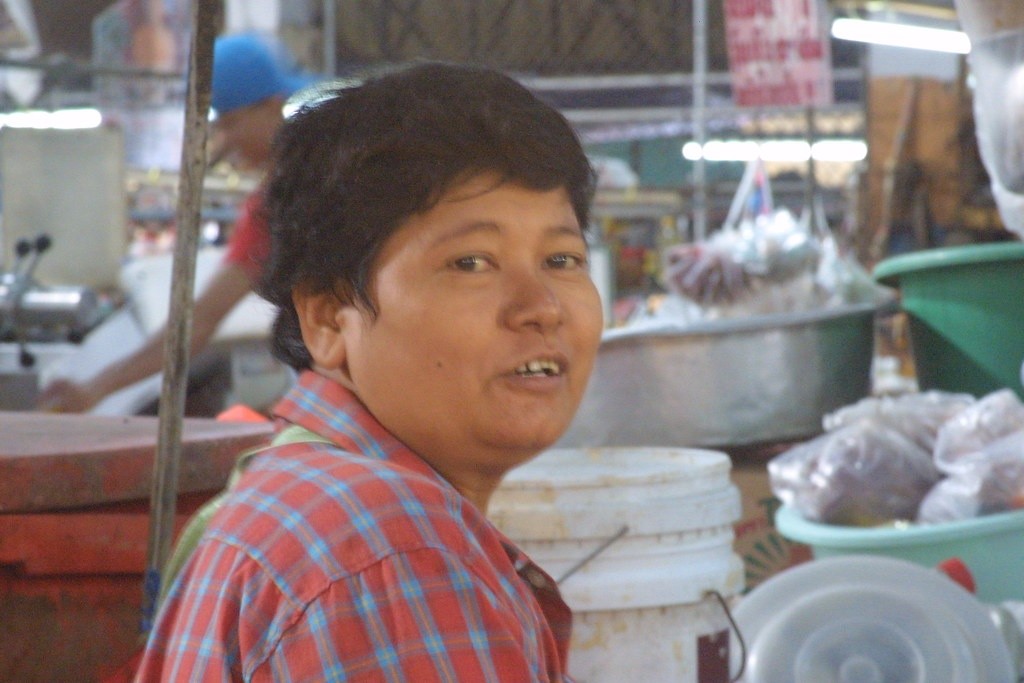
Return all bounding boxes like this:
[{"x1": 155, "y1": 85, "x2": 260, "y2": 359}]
[
  {"x1": 113, "y1": 59, "x2": 603, "y2": 683},
  {"x1": 48, "y1": 37, "x2": 310, "y2": 414}
]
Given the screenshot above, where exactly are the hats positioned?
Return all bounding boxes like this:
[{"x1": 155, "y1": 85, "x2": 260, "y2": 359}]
[{"x1": 212, "y1": 29, "x2": 318, "y2": 110}]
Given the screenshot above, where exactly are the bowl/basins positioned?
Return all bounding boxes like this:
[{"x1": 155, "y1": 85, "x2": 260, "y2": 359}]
[{"x1": 553, "y1": 305, "x2": 877, "y2": 449}]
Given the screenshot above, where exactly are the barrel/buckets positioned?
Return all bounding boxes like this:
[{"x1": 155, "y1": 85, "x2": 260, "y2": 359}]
[
  {"x1": 487, "y1": 446, "x2": 746, "y2": 683},
  {"x1": 773, "y1": 503, "x2": 1024, "y2": 605},
  {"x1": 872, "y1": 242, "x2": 1023, "y2": 404}
]
